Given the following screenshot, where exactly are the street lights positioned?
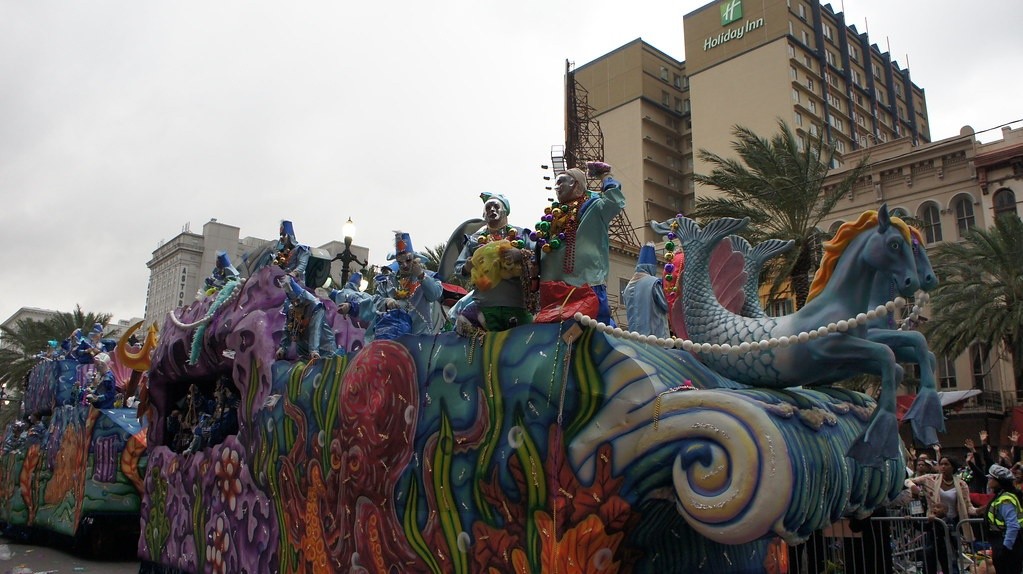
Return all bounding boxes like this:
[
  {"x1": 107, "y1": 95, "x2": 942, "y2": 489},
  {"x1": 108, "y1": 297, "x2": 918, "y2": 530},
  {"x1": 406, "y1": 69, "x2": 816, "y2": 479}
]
[
  {"x1": 330, "y1": 215, "x2": 368, "y2": 288},
  {"x1": 0, "y1": 384, "x2": 12, "y2": 411}
]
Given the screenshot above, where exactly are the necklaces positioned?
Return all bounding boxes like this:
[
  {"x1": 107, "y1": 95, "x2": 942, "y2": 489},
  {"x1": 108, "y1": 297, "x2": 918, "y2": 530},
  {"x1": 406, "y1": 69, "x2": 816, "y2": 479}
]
[
  {"x1": 664, "y1": 214, "x2": 682, "y2": 299},
  {"x1": 943, "y1": 479, "x2": 953, "y2": 485},
  {"x1": 477, "y1": 227, "x2": 527, "y2": 249},
  {"x1": 273, "y1": 249, "x2": 290, "y2": 264},
  {"x1": 529, "y1": 196, "x2": 587, "y2": 252}
]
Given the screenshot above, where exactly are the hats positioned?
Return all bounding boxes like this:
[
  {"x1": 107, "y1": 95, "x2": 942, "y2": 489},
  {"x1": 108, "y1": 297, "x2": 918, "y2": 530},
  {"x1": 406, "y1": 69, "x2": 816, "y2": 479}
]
[
  {"x1": 395, "y1": 233, "x2": 413, "y2": 256},
  {"x1": 106, "y1": 339, "x2": 116, "y2": 348},
  {"x1": 638, "y1": 245, "x2": 657, "y2": 265},
  {"x1": 32, "y1": 410, "x2": 42, "y2": 420},
  {"x1": 93, "y1": 322, "x2": 103, "y2": 333},
  {"x1": 280, "y1": 221, "x2": 294, "y2": 236},
  {"x1": 218, "y1": 253, "x2": 232, "y2": 268},
  {"x1": 15, "y1": 421, "x2": 23, "y2": 427},
  {"x1": 985, "y1": 464, "x2": 1016, "y2": 481},
  {"x1": 74, "y1": 328, "x2": 83, "y2": 341},
  {"x1": 558, "y1": 167, "x2": 588, "y2": 191},
  {"x1": 380, "y1": 266, "x2": 393, "y2": 272},
  {"x1": 281, "y1": 275, "x2": 303, "y2": 301},
  {"x1": 93, "y1": 352, "x2": 111, "y2": 366},
  {"x1": 47, "y1": 340, "x2": 58, "y2": 348},
  {"x1": 480, "y1": 193, "x2": 510, "y2": 217},
  {"x1": 60, "y1": 339, "x2": 71, "y2": 350},
  {"x1": 349, "y1": 272, "x2": 362, "y2": 287}
]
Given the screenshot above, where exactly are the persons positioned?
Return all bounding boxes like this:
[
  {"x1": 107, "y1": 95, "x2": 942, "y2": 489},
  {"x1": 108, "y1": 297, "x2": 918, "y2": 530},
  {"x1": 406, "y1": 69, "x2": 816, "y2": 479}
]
[
  {"x1": 447, "y1": 192, "x2": 539, "y2": 331},
  {"x1": 273, "y1": 277, "x2": 336, "y2": 360},
  {"x1": 529, "y1": 159, "x2": 626, "y2": 328},
  {"x1": 268, "y1": 220, "x2": 309, "y2": 280},
  {"x1": 848, "y1": 430, "x2": 1023, "y2": 574},
  {"x1": 36, "y1": 323, "x2": 115, "y2": 409},
  {"x1": 323, "y1": 232, "x2": 442, "y2": 343},
  {"x1": 623, "y1": 245, "x2": 669, "y2": 339},
  {"x1": 167, "y1": 376, "x2": 235, "y2": 453},
  {"x1": 211, "y1": 251, "x2": 239, "y2": 280}
]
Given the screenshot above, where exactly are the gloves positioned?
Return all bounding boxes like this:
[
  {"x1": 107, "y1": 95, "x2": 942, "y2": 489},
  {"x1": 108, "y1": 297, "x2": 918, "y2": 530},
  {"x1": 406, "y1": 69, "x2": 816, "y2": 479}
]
[{"x1": 587, "y1": 160, "x2": 612, "y2": 177}]
